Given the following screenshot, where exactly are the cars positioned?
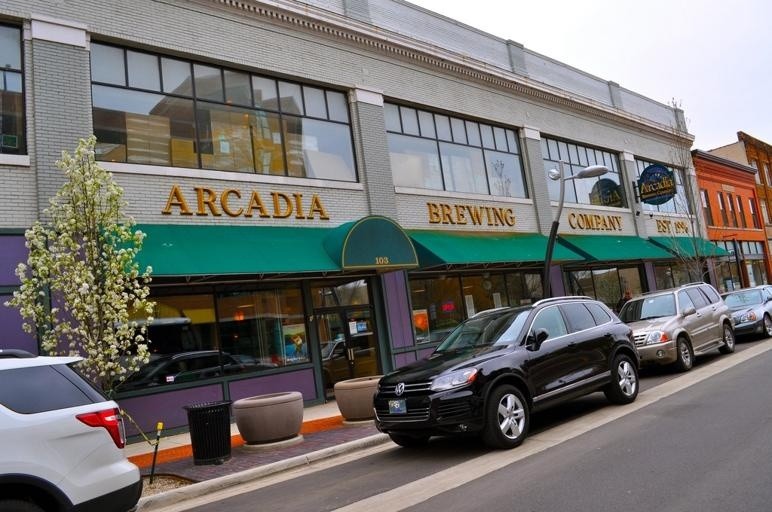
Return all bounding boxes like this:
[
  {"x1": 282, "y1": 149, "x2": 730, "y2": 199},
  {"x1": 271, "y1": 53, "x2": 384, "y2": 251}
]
[{"x1": 720, "y1": 284, "x2": 772, "y2": 338}]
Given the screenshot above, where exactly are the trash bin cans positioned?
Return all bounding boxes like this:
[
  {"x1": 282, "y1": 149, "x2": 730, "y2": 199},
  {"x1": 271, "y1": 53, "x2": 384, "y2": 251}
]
[{"x1": 182, "y1": 400, "x2": 233, "y2": 465}]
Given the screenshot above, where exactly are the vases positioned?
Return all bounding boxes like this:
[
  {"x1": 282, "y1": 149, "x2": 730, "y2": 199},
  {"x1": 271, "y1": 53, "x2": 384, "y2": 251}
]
[
  {"x1": 332, "y1": 374, "x2": 387, "y2": 424},
  {"x1": 234, "y1": 391, "x2": 305, "y2": 451}
]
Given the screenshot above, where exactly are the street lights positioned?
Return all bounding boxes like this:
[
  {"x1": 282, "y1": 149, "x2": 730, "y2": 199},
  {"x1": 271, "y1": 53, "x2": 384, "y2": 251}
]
[{"x1": 542, "y1": 159, "x2": 609, "y2": 298}]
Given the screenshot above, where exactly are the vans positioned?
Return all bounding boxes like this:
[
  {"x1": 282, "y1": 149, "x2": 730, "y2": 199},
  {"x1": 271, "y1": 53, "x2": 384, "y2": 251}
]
[
  {"x1": 616, "y1": 282, "x2": 736, "y2": 371},
  {"x1": 277, "y1": 330, "x2": 381, "y2": 389},
  {"x1": 0, "y1": 347, "x2": 143, "y2": 511},
  {"x1": 371, "y1": 296, "x2": 641, "y2": 450},
  {"x1": 113, "y1": 314, "x2": 283, "y2": 396}
]
